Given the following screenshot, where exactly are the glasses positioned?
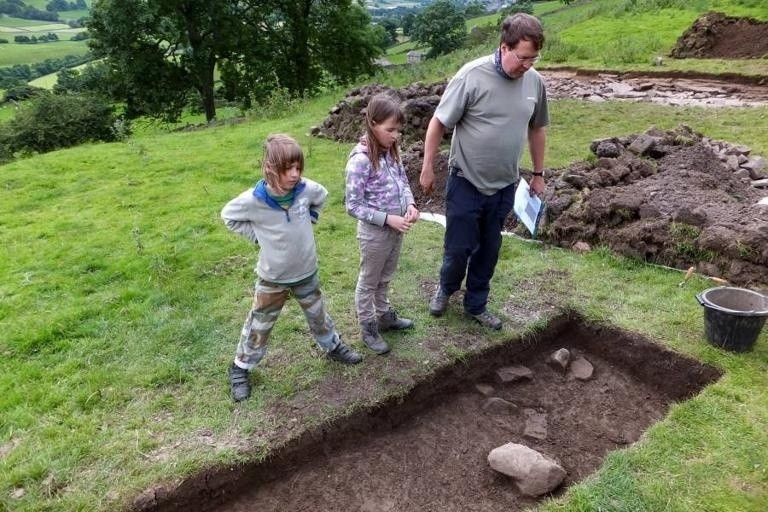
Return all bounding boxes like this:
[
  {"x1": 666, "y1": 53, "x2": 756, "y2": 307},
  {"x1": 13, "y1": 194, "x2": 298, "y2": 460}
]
[{"x1": 512, "y1": 50, "x2": 541, "y2": 63}]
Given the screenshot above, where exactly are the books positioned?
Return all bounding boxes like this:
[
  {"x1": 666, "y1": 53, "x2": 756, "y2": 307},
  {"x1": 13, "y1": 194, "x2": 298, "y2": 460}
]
[{"x1": 512, "y1": 176, "x2": 546, "y2": 237}]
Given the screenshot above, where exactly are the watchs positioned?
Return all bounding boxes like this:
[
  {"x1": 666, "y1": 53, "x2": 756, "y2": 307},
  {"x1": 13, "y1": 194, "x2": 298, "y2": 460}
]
[{"x1": 532, "y1": 171, "x2": 545, "y2": 177}]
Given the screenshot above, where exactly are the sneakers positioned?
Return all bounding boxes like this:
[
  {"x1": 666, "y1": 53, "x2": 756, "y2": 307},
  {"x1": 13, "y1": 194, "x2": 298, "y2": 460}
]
[
  {"x1": 359, "y1": 321, "x2": 389, "y2": 354},
  {"x1": 377, "y1": 308, "x2": 412, "y2": 331},
  {"x1": 230, "y1": 362, "x2": 250, "y2": 401},
  {"x1": 327, "y1": 341, "x2": 360, "y2": 363},
  {"x1": 464, "y1": 308, "x2": 501, "y2": 329},
  {"x1": 428, "y1": 285, "x2": 449, "y2": 315}
]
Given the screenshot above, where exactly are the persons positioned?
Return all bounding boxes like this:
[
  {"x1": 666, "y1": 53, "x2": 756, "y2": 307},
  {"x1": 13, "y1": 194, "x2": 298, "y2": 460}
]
[
  {"x1": 418, "y1": 12, "x2": 552, "y2": 331},
  {"x1": 220, "y1": 132, "x2": 364, "y2": 403},
  {"x1": 343, "y1": 92, "x2": 421, "y2": 356}
]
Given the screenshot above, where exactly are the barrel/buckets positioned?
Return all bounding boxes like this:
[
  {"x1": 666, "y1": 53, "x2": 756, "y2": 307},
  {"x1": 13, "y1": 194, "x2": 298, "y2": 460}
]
[{"x1": 693, "y1": 286, "x2": 768, "y2": 352}]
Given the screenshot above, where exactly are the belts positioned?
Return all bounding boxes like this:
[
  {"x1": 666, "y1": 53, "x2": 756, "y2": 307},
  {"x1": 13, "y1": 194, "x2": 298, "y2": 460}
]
[{"x1": 449, "y1": 167, "x2": 464, "y2": 177}]
